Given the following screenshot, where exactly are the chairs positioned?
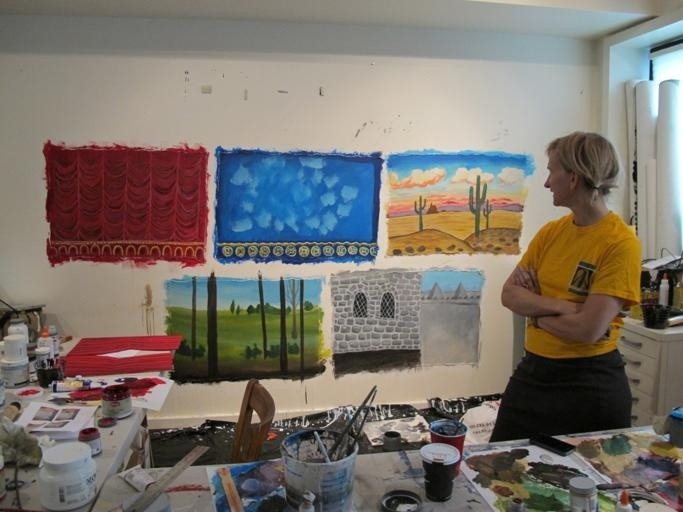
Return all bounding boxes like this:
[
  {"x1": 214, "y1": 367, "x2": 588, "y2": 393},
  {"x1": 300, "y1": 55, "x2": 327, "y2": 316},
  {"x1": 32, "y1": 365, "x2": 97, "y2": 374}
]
[{"x1": 225, "y1": 377, "x2": 276, "y2": 465}]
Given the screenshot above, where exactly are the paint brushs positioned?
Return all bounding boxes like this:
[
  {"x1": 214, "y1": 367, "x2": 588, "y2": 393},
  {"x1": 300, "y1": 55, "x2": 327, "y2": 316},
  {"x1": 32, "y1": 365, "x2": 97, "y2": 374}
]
[{"x1": 330, "y1": 386, "x2": 377, "y2": 456}]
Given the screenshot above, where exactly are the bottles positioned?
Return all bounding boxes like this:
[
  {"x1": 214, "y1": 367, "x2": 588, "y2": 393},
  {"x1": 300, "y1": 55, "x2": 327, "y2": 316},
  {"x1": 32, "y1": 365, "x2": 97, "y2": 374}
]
[
  {"x1": 569, "y1": 477, "x2": 598, "y2": 512},
  {"x1": 659, "y1": 272, "x2": 671, "y2": 311},
  {"x1": 0, "y1": 318, "x2": 60, "y2": 389},
  {"x1": 36, "y1": 385, "x2": 133, "y2": 511},
  {"x1": 1, "y1": 401, "x2": 22, "y2": 420},
  {"x1": 616, "y1": 491, "x2": 632, "y2": 512}
]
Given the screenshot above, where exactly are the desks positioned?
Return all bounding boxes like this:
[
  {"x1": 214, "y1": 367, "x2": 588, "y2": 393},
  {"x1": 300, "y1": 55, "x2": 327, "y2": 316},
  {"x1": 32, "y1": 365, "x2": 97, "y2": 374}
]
[
  {"x1": 0, "y1": 336, "x2": 180, "y2": 512},
  {"x1": 98, "y1": 425, "x2": 682, "y2": 512}
]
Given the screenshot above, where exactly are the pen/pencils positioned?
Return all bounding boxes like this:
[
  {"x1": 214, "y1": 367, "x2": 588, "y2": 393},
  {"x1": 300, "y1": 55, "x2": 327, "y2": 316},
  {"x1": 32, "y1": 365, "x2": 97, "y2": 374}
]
[
  {"x1": 280, "y1": 432, "x2": 331, "y2": 463},
  {"x1": 35, "y1": 357, "x2": 57, "y2": 367}
]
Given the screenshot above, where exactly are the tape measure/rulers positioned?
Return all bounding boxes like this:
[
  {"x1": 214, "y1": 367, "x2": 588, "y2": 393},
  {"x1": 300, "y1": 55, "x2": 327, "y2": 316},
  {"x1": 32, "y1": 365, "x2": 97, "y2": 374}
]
[
  {"x1": 122, "y1": 444, "x2": 210, "y2": 512},
  {"x1": 217, "y1": 466, "x2": 245, "y2": 511}
]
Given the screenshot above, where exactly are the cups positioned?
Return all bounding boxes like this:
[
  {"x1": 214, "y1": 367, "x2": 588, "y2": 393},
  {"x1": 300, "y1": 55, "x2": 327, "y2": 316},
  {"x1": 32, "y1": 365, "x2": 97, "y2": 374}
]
[
  {"x1": 419, "y1": 418, "x2": 468, "y2": 501},
  {"x1": 641, "y1": 304, "x2": 671, "y2": 330}
]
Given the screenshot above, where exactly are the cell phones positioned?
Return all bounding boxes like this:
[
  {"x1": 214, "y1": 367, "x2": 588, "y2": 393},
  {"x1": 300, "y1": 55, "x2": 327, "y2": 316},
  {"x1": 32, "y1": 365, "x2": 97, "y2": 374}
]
[{"x1": 530, "y1": 432, "x2": 576, "y2": 456}]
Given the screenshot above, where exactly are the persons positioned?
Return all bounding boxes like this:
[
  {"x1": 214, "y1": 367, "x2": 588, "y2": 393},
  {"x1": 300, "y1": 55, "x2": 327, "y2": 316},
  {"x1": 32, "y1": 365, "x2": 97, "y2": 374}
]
[{"x1": 489, "y1": 131, "x2": 643, "y2": 444}]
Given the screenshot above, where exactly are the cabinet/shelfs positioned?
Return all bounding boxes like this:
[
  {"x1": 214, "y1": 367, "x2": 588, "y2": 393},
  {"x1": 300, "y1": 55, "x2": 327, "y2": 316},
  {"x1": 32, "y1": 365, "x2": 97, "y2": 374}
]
[{"x1": 612, "y1": 317, "x2": 683, "y2": 433}]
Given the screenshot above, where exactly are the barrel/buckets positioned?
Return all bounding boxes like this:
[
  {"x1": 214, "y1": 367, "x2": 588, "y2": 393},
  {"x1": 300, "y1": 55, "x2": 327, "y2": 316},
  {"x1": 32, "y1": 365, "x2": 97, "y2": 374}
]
[{"x1": 280, "y1": 431, "x2": 358, "y2": 511}]
